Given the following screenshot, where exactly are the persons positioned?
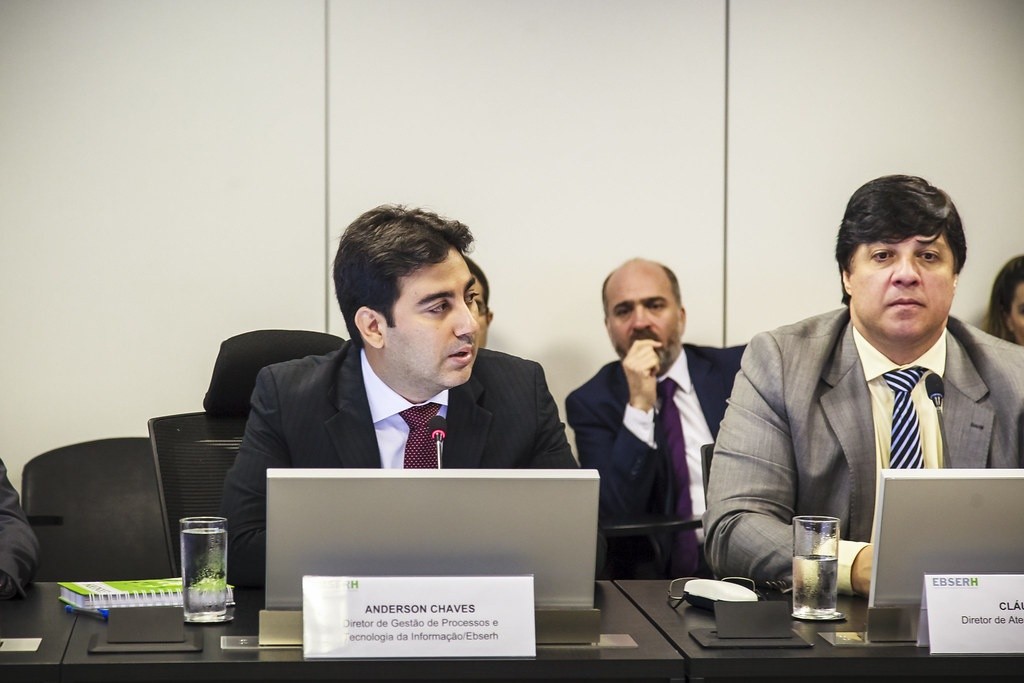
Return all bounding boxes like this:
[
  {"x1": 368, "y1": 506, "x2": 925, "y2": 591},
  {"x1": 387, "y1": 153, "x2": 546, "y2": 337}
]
[
  {"x1": 701, "y1": 178, "x2": 1023, "y2": 597},
  {"x1": 218, "y1": 204, "x2": 580, "y2": 589},
  {"x1": 565, "y1": 258, "x2": 748, "y2": 579},
  {"x1": 0, "y1": 457, "x2": 43, "y2": 605},
  {"x1": 464, "y1": 254, "x2": 494, "y2": 349},
  {"x1": 981, "y1": 255, "x2": 1024, "y2": 347}
]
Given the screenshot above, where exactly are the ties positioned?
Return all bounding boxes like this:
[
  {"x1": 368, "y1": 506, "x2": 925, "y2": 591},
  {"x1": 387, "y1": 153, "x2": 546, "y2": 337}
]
[
  {"x1": 398, "y1": 402, "x2": 443, "y2": 470},
  {"x1": 881, "y1": 367, "x2": 929, "y2": 469},
  {"x1": 656, "y1": 377, "x2": 699, "y2": 579}
]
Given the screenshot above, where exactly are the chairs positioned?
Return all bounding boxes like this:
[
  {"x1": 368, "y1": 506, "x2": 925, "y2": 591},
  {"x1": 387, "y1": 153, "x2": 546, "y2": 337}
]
[{"x1": 18, "y1": 410, "x2": 725, "y2": 583}]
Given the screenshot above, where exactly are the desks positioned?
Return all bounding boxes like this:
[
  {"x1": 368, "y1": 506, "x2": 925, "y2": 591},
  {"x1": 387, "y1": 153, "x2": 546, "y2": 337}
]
[{"x1": 0, "y1": 579, "x2": 1024, "y2": 683}]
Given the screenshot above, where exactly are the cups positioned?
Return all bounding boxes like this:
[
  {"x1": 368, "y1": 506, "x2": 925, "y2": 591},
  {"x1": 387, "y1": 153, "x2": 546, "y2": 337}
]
[
  {"x1": 179, "y1": 517, "x2": 228, "y2": 621},
  {"x1": 792, "y1": 515, "x2": 840, "y2": 619}
]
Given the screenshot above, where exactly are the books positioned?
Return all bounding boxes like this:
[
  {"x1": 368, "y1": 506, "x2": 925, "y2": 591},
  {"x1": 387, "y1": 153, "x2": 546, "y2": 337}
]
[{"x1": 57, "y1": 575, "x2": 235, "y2": 611}]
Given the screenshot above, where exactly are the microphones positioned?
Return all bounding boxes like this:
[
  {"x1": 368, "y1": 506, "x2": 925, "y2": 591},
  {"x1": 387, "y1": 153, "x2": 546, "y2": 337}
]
[
  {"x1": 924, "y1": 373, "x2": 952, "y2": 468},
  {"x1": 429, "y1": 416, "x2": 447, "y2": 470}
]
